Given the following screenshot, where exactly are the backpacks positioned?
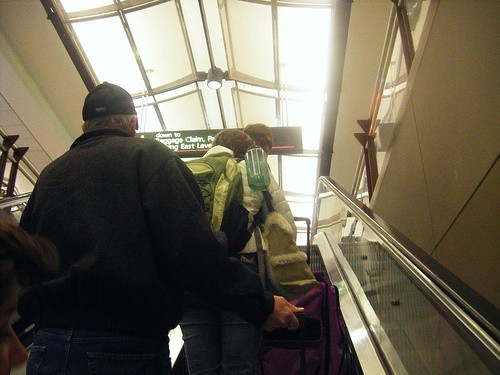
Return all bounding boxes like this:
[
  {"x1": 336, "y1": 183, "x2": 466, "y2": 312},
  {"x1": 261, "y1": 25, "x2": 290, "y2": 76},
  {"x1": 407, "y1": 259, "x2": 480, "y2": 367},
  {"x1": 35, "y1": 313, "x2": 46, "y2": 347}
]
[{"x1": 182, "y1": 155, "x2": 275, "y2": 295}]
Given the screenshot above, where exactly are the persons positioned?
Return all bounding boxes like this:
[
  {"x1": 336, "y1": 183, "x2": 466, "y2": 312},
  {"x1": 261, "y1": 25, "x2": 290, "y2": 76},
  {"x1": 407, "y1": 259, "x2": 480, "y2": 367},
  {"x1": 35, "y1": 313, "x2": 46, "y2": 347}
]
[
  {"x1": 178, "y1": 123, "x2": 298, "y2": 375},
  {"x1": 16, "y1": 82, "x2": 304, "y2": 375},
  {"x1": 0, "y1": 208, "x2": 61, "y2": 375}
]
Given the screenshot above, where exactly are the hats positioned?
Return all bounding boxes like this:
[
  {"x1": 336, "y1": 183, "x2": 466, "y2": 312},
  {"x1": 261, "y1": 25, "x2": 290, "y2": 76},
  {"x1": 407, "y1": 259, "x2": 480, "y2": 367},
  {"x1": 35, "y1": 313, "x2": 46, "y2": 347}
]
[{"x1": 82, "y1": 81, "x2": 138, "y2": 130}]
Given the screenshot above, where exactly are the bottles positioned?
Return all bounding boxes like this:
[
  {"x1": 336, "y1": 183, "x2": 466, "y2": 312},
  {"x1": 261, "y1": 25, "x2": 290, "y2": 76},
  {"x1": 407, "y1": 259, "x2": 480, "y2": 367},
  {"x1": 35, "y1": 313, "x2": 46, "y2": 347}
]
[{"x1": 246, "y1": 145, "x2": 271, "y2": 191}]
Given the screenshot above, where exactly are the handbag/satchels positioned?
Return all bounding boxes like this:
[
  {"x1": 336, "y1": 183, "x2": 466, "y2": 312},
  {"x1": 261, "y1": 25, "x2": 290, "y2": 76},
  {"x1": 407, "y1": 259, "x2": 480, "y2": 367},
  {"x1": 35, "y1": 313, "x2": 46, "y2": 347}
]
[{"x1": 259, "y1": 277, "x2": 346, "y2": 374}]
